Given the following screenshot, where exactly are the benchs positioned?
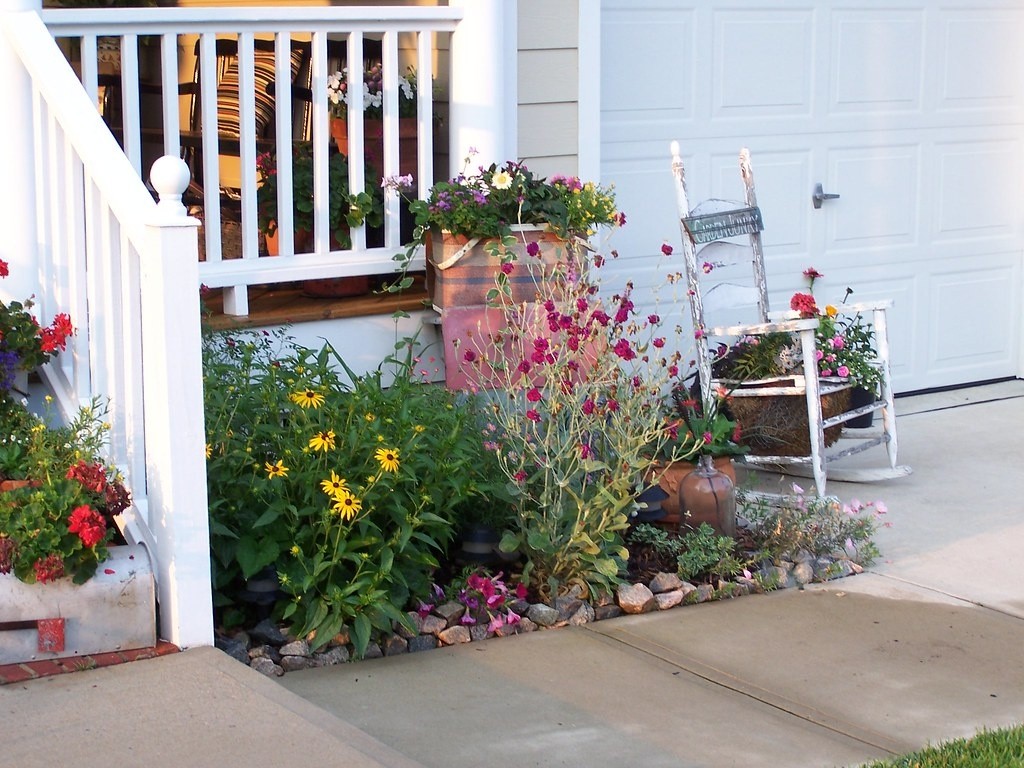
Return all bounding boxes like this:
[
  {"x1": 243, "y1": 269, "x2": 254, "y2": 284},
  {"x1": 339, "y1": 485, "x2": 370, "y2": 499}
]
[{"x1": 98, "y1": 39, "x2": 384, "y2": 223}]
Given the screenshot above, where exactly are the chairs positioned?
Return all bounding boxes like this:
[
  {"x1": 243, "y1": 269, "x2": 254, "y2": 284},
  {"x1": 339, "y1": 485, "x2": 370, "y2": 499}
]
[{"x1": 669, "y1": 139, "x2": 913, "y2": 509}]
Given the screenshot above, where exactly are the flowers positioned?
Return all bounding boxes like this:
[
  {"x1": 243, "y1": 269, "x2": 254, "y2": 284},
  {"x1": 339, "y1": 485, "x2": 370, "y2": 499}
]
[
  {"x1": 256, "y1": 134, "x2": 384, "y2": 249},
  {"x1": 707, "y1": 265, "x2": 889, "y2": 395},
  {"x1": 372, "y1": 146, "x2": 617, "y2": 296},
  {"x1": 634, "y1": 386, "x2": 753, "y2": 468},
  {"x1": 0, "y1": 259, "x2": 133, "y2": 586},
  {"x1": 328, "y1": 64, "x2": 447, "y2": 125}
]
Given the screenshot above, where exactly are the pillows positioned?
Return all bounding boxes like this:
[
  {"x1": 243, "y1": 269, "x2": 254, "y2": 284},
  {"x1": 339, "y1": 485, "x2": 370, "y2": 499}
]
[{"x1": 203, "y1": 48, "x2": 304, "y2": 140}]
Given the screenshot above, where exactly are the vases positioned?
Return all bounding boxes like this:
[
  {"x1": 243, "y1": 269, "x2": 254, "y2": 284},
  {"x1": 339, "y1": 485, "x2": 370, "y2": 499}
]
[
  {"x1": 1, "y1": 363, "x2": 28, "y2": 404},
  {"x1": 639, "y1": 452, "x2": 737, "y2": 536},
  {"x1": 430, "y1": 221, "x2": 600, "y2": 314},
  {"x1": 331, "y1": 117, "x2": 439, "y2": 189},
  {"x1": 726, "y1": 382, "x2": 851, "y2": 457},
  {"x1": 845, "y1": 382, "x2": 877, "y2": 428},
  {"x1": 264, "y1": 217, "x2": 370, "y2": 296}
]
